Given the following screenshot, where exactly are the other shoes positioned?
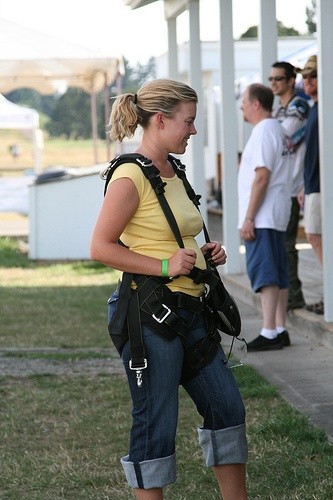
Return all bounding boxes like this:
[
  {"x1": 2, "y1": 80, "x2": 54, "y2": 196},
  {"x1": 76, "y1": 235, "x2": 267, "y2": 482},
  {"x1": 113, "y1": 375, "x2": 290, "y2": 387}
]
[
  {"x1": 306, "y1": 301, "x2": 323, "y2": 314},
  {"x1": 237, "y1": 329, "x2": 290, "y2": 352},
  {"x1": 285, "y1": 300, "x2": 305, "y2": 312}
]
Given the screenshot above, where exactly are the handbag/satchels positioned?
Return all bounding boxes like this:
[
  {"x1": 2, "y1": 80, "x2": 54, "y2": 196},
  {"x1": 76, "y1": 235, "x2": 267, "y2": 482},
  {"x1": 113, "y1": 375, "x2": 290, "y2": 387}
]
[{"x1": 205, "y1": 260, "x2": 241, "y2": 338}]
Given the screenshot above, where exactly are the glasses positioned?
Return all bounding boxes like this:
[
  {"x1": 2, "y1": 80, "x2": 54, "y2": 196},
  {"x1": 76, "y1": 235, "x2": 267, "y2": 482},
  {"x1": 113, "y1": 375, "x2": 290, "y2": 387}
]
[
  {"x1": 268, "y1": 74, "x2": 291, "y2": 81},
  {"x1": 302, "y1": 73, "x2": 317, "y2": 79}
]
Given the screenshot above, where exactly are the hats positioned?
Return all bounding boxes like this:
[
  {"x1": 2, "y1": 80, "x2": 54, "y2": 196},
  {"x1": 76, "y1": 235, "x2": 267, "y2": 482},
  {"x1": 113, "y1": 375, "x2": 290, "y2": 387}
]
[{"x1": 294, "y1": 55, "x2": 317, "y2": 74}]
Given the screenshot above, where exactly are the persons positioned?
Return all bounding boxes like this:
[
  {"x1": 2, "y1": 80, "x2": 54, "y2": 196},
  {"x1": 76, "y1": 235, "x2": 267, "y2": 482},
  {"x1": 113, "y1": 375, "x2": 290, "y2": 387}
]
[
  {"x1": 89, "y1": 77, "x2": 249, "y2": 500},
  {"x1": 235, "y1": 54, "x2": 325, "y2": 352}
]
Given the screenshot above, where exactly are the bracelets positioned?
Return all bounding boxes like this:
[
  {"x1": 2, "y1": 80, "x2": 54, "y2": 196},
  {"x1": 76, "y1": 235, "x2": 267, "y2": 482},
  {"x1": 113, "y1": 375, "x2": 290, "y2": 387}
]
[{"x1": 161, "y1": 258, "x2": 170, "y2": 278}]
